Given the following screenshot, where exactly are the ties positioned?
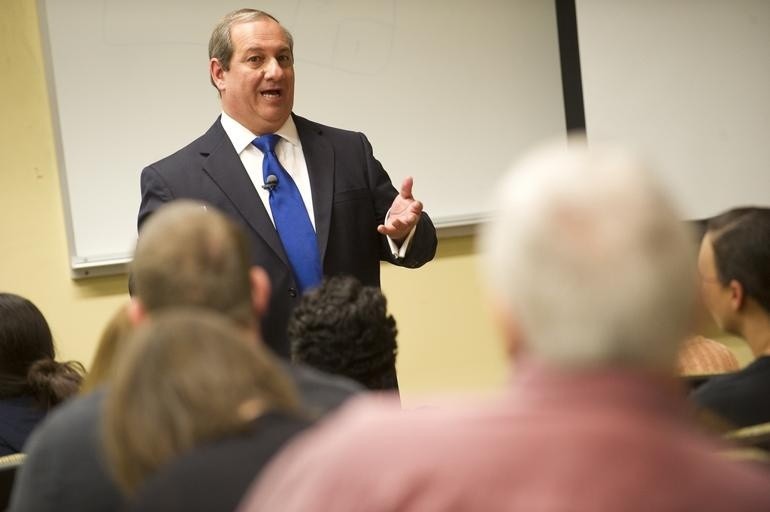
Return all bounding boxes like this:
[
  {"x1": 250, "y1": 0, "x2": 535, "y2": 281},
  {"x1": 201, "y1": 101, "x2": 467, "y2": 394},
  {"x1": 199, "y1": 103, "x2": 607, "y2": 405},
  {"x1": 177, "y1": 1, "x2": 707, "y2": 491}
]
[{"x1": 250, "y1": 136, "x2": 321, "y2": 290}]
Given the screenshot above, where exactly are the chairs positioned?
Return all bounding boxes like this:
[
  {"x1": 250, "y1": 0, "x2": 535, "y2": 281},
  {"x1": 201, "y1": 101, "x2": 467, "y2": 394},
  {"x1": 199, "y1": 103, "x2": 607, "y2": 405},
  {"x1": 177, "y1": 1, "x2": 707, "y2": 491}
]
[
  {"x1": 715, "y1": 422, "x2": 770, "y2": 464},
  {"x1": 0, "y1": 452, "x2": 27, "y2": 512}
]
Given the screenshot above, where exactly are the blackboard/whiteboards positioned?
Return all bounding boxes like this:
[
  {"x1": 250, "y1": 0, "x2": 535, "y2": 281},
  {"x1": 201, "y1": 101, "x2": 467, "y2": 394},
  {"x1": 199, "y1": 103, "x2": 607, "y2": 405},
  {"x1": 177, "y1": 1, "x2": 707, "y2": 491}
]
[
  {"x1": 38, "y1": 3, "x2": 573, "y2": 268},
  {"x1": 568, "y1": 3, "x2": 770, "y2": 232}
]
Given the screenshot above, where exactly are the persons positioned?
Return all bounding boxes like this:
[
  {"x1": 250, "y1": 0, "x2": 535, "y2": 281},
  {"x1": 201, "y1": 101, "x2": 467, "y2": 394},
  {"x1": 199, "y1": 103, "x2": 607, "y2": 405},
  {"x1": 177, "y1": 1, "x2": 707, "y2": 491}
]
[
  {"x1": 686, "y1": 205, "x2": 770, "y2": 452},
  {"x1": 102, "y1": 311, "x2": 316, "y2": 509},
  {"x1": 676, "y1": 332, "x2": 740, "y2": 375},
  {"x1": 287, "y1": 273, "x2": 399, "y2": 392},
  {"x1": 1, "y1": 291, "x2": 89, "y2": 456},
  {"x1": 238, "y1": 128, "x2": 770, "y2": 509},
  {"x1": 11, "y1": 199, "x2": 369, "y2": 508},
  {"x1": 130, "y1": 5, "x2": 438, "y2": 394}
]
